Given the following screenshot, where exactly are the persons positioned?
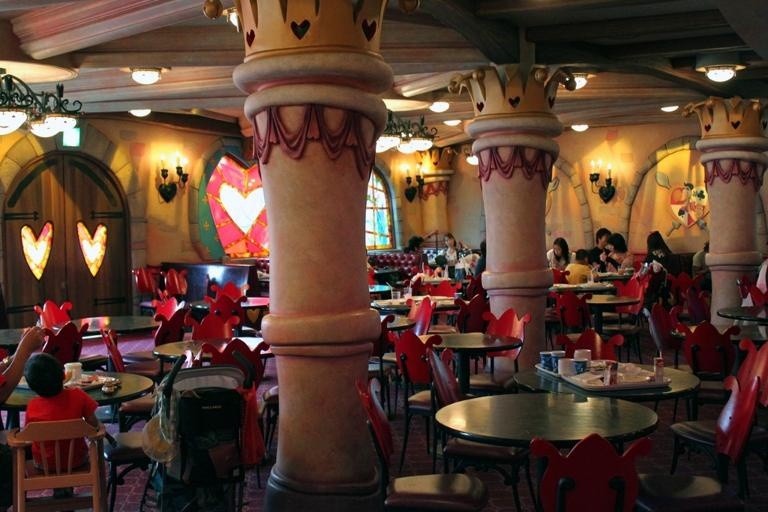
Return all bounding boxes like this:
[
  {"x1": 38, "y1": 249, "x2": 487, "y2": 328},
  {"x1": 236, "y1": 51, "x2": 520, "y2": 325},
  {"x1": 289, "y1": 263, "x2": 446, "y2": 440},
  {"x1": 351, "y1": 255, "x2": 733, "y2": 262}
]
[
  {"x1": 546, "y1": 228, "x2": 709, "y2": 284},
  {"x1": 24, "y1": 352, "x2": 99, "y2": 498},
  {"x1": 406, "y1": 233, "x2": 486, "y2": 283},
  {"x1": 0, "y1": 325, "x2": 45, "y2": 512}
]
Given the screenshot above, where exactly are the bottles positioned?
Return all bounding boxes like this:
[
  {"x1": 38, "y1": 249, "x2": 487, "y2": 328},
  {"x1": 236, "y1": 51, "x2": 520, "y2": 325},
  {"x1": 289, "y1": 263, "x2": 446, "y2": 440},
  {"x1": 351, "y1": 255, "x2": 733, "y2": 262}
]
[
  {"x1": 654, "y1": 358, "x2": 664, "y2": 382},
  {"x1": 603, "y1": 358, "x2": 618, "y2": 385}
]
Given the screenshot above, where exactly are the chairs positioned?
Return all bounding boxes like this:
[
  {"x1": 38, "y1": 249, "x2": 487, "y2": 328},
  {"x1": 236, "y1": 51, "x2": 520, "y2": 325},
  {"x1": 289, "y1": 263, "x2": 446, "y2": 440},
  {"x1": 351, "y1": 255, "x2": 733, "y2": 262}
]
[{"x1": 0, "y1": 252, "x2": 768, "y2": 512}]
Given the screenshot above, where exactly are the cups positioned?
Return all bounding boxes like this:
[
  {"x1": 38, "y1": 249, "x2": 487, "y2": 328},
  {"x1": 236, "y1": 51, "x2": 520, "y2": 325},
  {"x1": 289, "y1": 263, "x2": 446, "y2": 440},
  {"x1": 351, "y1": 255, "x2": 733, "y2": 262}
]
[
  {"x1": 455, "y1": 293, "x2": 463, "y2": 301},
  {"x1": 403, "y1": 286, "x2": 412, "y2": 300},
  {"x1": 539, "y1": 348, "x2": 593, "y2": 375},
  {"x1": 392, "y1": 291, "x2": 400, "y2": 302},
  {"x1": 617, "y1": 265, "x2": 625, "y2": 275},
  {"x1": 63, "y1": 362, "x2": 82, "y2": 382}
]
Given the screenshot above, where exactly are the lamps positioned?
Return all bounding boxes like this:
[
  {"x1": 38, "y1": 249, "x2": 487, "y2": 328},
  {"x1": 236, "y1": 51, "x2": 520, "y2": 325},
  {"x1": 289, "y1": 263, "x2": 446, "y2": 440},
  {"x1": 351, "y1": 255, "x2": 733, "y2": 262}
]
[
  {"x1": 401, "y1": 162, "x2": 427, "y2": 203},
  {"x1": 129, "y1": 108, "x2": 151, "y2": 118},
  {"x1": 443, "y1": 120, "x2": 462, "y2": 127},
  {"x1": 571, "y1": 125, "x2": 589, "y2": 131},
  {"x1": 695, "y1": 54, "x2": 747, "y2": 84},
  {"x1": 0, "y1": 68, "x2": 82, "y2": 138},
  {"x1": 466, "y1": 155, "x2": 479, "y2": 166},
  {"x1": 376, "y1": 110, "x2": 439, "y2": 153},
  {"x1": 429, "y1": 102, "x2": 449, "y2": 113},
  {"x1": 565, "y1": 73, "x2": 587, "y2": 90},
  {"x1": 159, "y1": 151, "x2": 188, "y2": 203},
  {"x1": 589, "y1": 159, "x2": 616, "y2": 203},
  {"x1": 131, "y1": 68, "x2": 160, "y2": 85},
  {"x1": 661, "y1": 106, "x2": 679, "y2": 113}
]
